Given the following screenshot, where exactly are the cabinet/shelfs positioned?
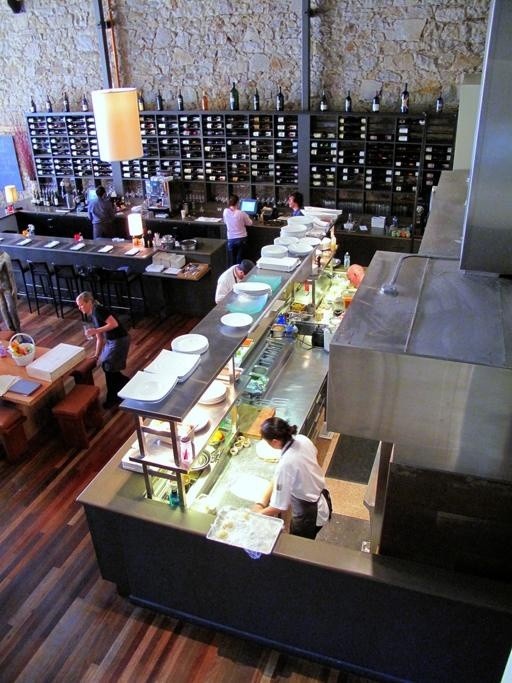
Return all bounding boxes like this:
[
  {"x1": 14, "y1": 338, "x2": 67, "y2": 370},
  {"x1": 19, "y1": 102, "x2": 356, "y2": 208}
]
[
  {"x1": 21, "y1": 109, "x2": 123, "y2": 224},
  {"x1": 333, "y1": 232, "x2": 421, "y2": 267},
  {"x1": 118, "y1": 223, "x2": 338, "y2": 509},
  {"x1": 15, "y1": 211, "x2": 94, "y2": 239},
  {"x1": 124, "y1": 108, "x2": 311, "y2": 223},
  {"x1": 144, "y1": 218, "x2": 224, "y2": 243},
  {"x1": 311, "y1": 107, "x2": 459, "y2": 222},
  {"x1": 243, "y1": 225, "x2": 281, "y2": 263}
]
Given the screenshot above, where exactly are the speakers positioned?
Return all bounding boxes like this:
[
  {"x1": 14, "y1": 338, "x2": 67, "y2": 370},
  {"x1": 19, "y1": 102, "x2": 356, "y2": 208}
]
[{"x1": 7, "y1": 0, "x2": 21, "y2": 13}]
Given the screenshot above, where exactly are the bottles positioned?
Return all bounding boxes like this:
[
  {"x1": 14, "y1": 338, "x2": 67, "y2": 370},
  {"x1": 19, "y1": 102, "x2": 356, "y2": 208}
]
[
  {"x1": 81, "y1": 93, "x2": 87, "y2": 112},
  {"x1": 46, "y1": 95, "x2": 52, "y2": 113},
  {"x1": 320, "y1": 90, "x2": 328, "y2": 113},
  {"x1": 276, "y1": 86, "x2": 285, "y2": 111},
  {"x1": 0, "y1": 342, "x2": 7, "y2": 358},
  {"x1": 331, "y1": 234, "x2": 337, "y2": 253},
  {"x1": 177, "y1": 424, "x2": 196, "y2": 468},
  {"x1": 169, "y1": 480, "x2": 180, "y2": 510},
  {"x1": 372, "y1": 91, "x2": 379, "y2": 112},
  {"x1": 63, "y1": 93, "x2": 69, "y2": 112},
  {"x1": 384, "y1": 223, "x2": 412, "y2": 238},
  {"x1": 146, "y1": 229, "x2": 175, "y2": 249},
  {"x1": 401, "y1": 83, "x2": 409, "y2": 112},
  {"x1": 156, "y1": 90, "x2": 163, "y2": 111},
  {"x1": 344, "y1": 90, "x2": 352, "y2": 113},
  {"x1": 182, "y1": 261, "x2": 200, "y2": 277},
  {"x1": 178, "y1": 89, "x2": 184, "y2": 111},
  {"x1": 310, "y1": 325, "x2": 324, "y2": 348},
  {"x1": 324, "y1": 308, "x2": 343, "y2": 353},
  {"x1": 253, "y1": 87, "x2": 260, "y2": 110},
  {"x1": 229, "y1": 82, "x2": 239, "y2": 111},
  {"x1": 26, "y1": 113, "x2": 455, "y2": 235},
  {"x1": 314, "y1": 307, "x2": 324, "y2": 320},
  {"x1": 30, "y1": 96, "x2": 36, "y2": 114},
  {"x1": 304, "y1": 280, "x2": 310, "y2": 291},
  {"x1": 271, "y1": 311, "x2": 299, "y2": 340},
  {"x1": 343, "y1": 251, "x2": 351, "y2": 268},
  {"x1": 436, "y1": 92, "x2": 444, "y2": 113},
  {"x1": 138, "y1": 91, "x2": 145, "y2": 111},
  {"x1": 201, "y1": 91, "x2": 209, "y2": 111}
]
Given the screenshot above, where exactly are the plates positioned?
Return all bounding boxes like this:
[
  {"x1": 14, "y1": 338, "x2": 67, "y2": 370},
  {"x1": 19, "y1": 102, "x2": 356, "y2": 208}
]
[
  {"x1": 261, "y1": 215, "x2": 329, "y2": 273},
  {"x1": 171, "y1": 333, "x2": 209, "y2": 354},
  {"x1": 117, "y1": 348, "x2": 202, "y2": 404},
  {"x1": 181, "y1": 406, "x2": 210, "y2": 433},
  {"x1": 145, "y1": 251, "x2": 185, "y2": 275},
  {"x1": 0, "y1": 237, "x2": 142, "y2": 255},
  {"x1": 198, "y1": 379, "x2": 227, "y2": 405},
  {"x1": 220, "y1": 312, "x2": 253, "y2": 328}
]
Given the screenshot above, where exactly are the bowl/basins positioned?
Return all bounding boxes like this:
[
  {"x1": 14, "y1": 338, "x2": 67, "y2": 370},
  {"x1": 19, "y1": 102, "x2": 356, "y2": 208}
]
[
  {"x1": 190, "y1": 447, "x2": 212, "y2": 472},
  {"x1": 179, "y1": 238, "x2": 197, "y2": 250},
  {"x1": 220, "y1": 325, "x2": 253, "y2": 338},
  {"x1": 232, "y1": 281, "x2": 272, "y2": 298},
  {"x1": 331, "y1": 257, "x2": 341, "y2": 269}
]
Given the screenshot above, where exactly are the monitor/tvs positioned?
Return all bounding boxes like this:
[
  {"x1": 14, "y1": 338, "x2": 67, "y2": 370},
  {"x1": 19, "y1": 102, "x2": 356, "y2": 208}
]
[
  {"x1": 240, "y1": 199, "x2": 258, "y2": 216},
  {"x1": 87, "y1": 188, "x2": 96, "y2": 200}
]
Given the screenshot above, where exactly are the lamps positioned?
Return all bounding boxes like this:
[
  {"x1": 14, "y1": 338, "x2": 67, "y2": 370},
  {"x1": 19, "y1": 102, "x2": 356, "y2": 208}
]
[
  {"x1": 87, "y1": 0, "x2": 146, "y2": 165},
  {"x1": 126, "y1": 213, "x2": 144, "y2": 244},
  {"x1": 4, "y1": 184, "x2": 22, "y2": 210}
]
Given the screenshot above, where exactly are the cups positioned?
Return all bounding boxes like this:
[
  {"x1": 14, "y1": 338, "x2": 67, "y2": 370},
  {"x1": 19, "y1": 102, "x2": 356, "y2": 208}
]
[
  {"x1": 181, "y1": 473, "x2": 192, "y2": 494},
  {"x1": 229, "y1": 435, "x2": 251, "y2": 456},
  {"x1": 342, "y1": 293, "x2": 353, "y2": 308}
]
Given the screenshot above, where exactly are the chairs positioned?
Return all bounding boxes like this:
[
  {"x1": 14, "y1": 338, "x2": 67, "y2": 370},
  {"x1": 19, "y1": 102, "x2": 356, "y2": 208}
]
[{"x1": 8, "y1": 258, "x2": 135, "y2": 319}]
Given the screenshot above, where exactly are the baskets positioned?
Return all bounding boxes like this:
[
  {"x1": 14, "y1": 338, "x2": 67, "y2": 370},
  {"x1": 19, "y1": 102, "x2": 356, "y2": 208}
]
[{"x1": 9, "y1": 333, "x2": 36, "y2": 367}]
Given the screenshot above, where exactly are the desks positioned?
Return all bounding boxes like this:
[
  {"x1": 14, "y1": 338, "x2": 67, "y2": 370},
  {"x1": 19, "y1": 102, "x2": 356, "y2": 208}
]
[
  {"x1": 0, "y1": 329, "x2": 87, "y2": 441},
  {"x1": 141, "y1": 260, "x2": 212, "y2": 281}
]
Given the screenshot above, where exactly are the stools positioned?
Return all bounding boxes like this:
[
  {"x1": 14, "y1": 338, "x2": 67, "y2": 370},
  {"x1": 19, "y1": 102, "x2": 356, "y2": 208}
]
[
  {"x1": 0, "y1": 405, "x2": 28, "y2": 459},
  {"x1": 51, "y1": 382, "x2": 105, "y2": 449},
  {"x1": 74, "y1": 353, "x2": 104, "y2": 389}
]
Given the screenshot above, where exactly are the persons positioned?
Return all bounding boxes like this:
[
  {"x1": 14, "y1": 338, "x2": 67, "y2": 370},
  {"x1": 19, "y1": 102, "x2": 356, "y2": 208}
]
[
  {"x1": 0, "y1": 249, "x2": 20, "y2": 334},
  {"x1": 255, "y1": 417, "x2": 333, "y2": 540},
  {"x1": 215, "y1": 259, "x2": 255, "y2": 307},
  {"x1": 87, "y1": 186, "x2": 114, "y2": 241},
  {"x1": 288, "y1": 192, "x2": 304, "y2": 217},
  {"x1": 346, "y1": 264, "x2": 365, "y2": 289},
  {"x1": 75, "y1": 292, "x2": 130, "y2": 410},
  {"x1": 222, "y1": 194, "x2": 253, "y2": 266}
]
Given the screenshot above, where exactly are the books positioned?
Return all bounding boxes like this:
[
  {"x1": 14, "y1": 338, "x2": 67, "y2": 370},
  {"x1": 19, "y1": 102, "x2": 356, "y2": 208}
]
[{"x1": 8, "y1": 379, "x2": 42, "y2": 396}]
